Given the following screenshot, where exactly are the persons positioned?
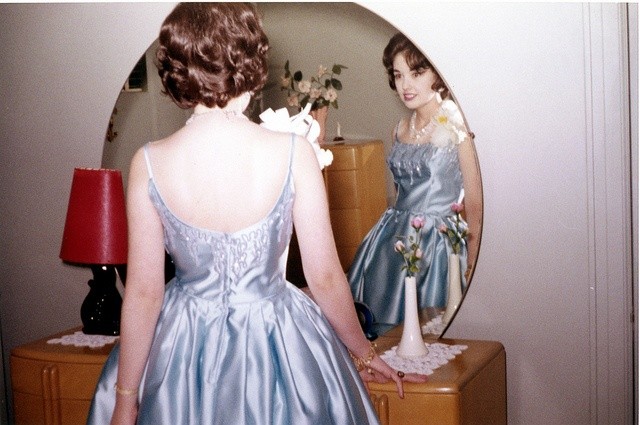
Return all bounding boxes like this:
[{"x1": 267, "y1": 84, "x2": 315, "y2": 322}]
[
  {"x1": 345, "y1": 32, "x2": 483, "y2": 337},
  {"x1": 86, "y1": 2, "x2": 428, "y2": 424}
]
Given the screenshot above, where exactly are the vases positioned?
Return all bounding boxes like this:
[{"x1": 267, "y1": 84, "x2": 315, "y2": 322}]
[
  {"x1": 396, "y1": 275, "x2": 429, "y2": 360},
  {"x1": 302, "y1": 101, "x2": 330, "y2": 143},
  {"x1": 443, "y1": 253, "x2": 463, "y2": 327}
]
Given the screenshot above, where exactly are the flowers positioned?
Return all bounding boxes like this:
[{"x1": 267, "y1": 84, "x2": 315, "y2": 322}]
[
  {"x1": 393, "y1": 212, "x2": 426, "y2": 275},
  {"x1": 280, "y1": 58, "x2": 349, "y2": 110},
  {"x1": 433, "y1": 203, "x2": 474, "y2": 254}
]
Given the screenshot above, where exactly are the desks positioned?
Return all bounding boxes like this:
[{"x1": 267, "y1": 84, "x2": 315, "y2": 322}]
[
  {"x1": 7, "y1": 325, "x2": 114, "y2": 425},
  {"x1": 359, "y1": 335, "x2": 507, "y2": 425}
]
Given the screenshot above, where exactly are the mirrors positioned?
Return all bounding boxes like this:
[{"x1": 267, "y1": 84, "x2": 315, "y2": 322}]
[{"x1": 100, "y1": 3, "x2": 482, "y2": 340}]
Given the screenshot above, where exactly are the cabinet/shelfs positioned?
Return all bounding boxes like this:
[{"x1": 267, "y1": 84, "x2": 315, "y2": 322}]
[{"x1": 319, "y1": 138, "x2": 386, "y2": 275}]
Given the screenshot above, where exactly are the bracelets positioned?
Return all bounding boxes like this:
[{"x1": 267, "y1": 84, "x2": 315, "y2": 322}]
[
  {"x1": 464, "y1": 265, "x2": 472, "y2": 281},
  {"x1": 347, "y1": 342, "x2": 377, "y2": 377},
  {"x1": 114, "y1": 383, "x2": 138, "y2": 396}
]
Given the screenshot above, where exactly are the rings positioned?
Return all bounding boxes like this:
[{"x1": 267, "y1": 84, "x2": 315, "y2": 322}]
[{"x1": 397, "y1": 371, "x2": 406, "y2": 381}]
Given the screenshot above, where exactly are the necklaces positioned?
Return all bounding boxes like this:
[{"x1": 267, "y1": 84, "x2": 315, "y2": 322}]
[
  {"x1": 409, "y1": 110, "x2": 445, "y2": 141},
  {"x1": 185, "y1": 110, "x2": 248, "y2": 125}
]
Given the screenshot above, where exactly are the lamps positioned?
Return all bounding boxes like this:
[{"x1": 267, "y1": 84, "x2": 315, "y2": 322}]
[{"x1": 58, "y1": 166, "x2": 127, "y2": 336}]
